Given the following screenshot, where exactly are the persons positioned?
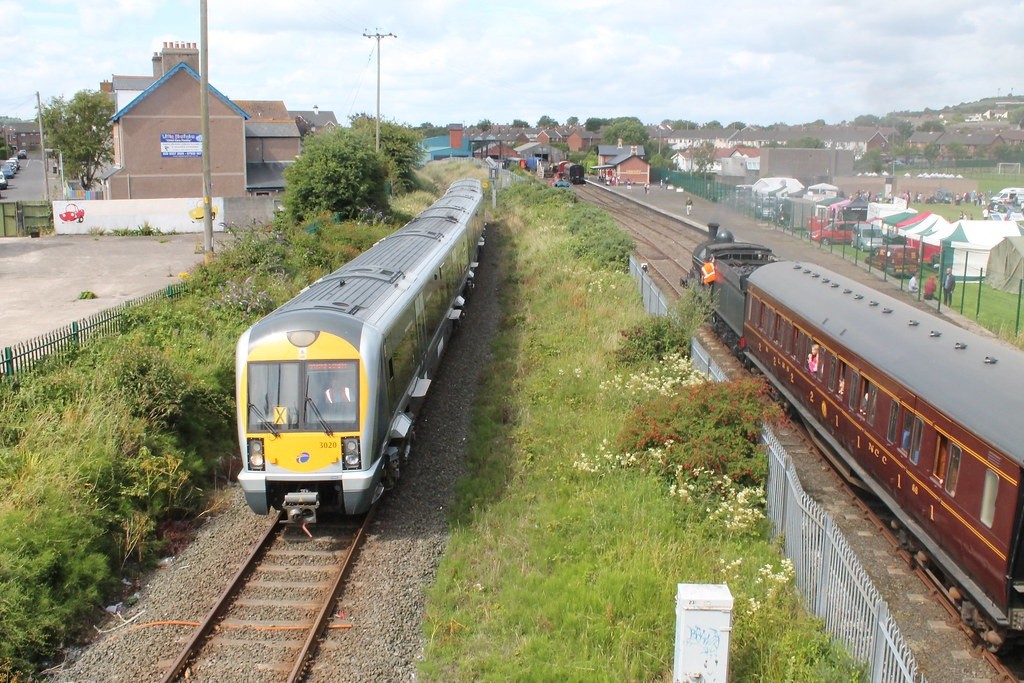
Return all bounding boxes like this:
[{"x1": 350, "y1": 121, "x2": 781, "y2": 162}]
[
  {"x1": 644, "y1": 183, "x2": 650, "y2": 192},
  {"x1": 942, "y1": 268, "x2": 955, "y2": 307},
  {"x1": 954, "y1": 191, "x2": 1024, "y2": 221},
  {"x1": 849, "y1": 189, "x2": 955, "y2": 205},
  {"x1": 685, "y1": 196, "x2": 693, "y2": 218},
  {"x1": 324, "y1": 375, "x2": 355, "y2": 404},
  {"x1": 908, "y1": 271, "x2": 921, "y2": 293},
  {"x1": 660, "y1": 175, "x2": 670, "y2": 189},
  {"x1": 598, "y1": 169, "x2": 632, "y2": 187},
  {"x1": 808, "y1": 345, "x2": 820, "y2": 373},
  {"x1": 923, "y1": 276, "x2": 939, "y2": 302}
]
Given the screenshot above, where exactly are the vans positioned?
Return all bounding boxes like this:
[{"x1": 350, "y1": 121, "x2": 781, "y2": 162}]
[
  {"x1": 850, "y1": 224, "x2": 885, "y2": 251},
  {"x1": 865, "y1": 244, "x2": 916, "y2": 278},
  {"x1": 990, "y1": 187, "x2": 1024, "y2": 204}
]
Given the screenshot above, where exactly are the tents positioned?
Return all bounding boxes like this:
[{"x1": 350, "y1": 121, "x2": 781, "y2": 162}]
[{"x1": 871, "y1": 211, "x2": 1024, "y2": 296}]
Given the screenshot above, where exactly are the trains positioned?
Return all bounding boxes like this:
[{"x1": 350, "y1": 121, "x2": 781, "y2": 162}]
[
  {"x1": 237, "y1": 178, "x2": 485, "y2": 533},
  {"x1": 502, "y1": 157, "x2": 585, "y2": 185},
  {"x1": 681, "y1": 223, "x2": 1023, "y2": 654}
]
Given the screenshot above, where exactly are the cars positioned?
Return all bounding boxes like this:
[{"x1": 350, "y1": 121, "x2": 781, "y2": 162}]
[
  {"x1": 811, "y1": 222, "x2": 853, "y2": 246},
  {"x1": 754, "y1": 200, "x2": 775, "y2": 220},
  {"x1": 0, "y1": 149, "x2": 26, "y2": 189}
]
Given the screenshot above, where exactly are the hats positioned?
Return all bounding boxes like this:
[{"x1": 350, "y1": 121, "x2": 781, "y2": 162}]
[{"x1": 929, "y1": 275, "x2": 935, "y2": 280}]
